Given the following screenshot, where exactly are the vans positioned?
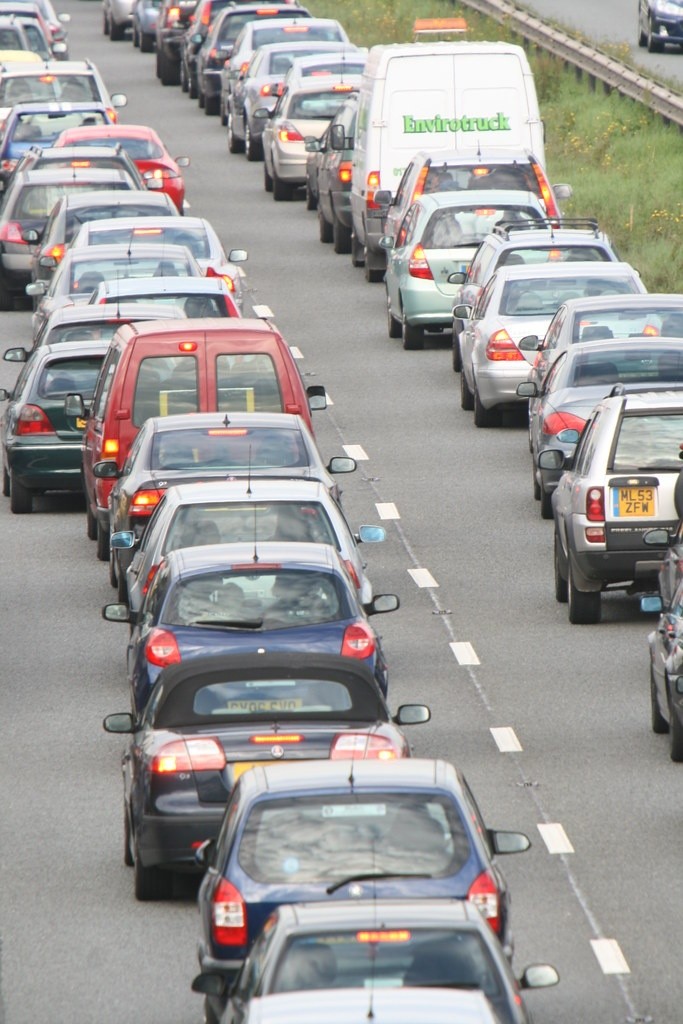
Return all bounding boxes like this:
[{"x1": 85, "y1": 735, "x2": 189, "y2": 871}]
[{"x1": 62, "y1": 316, "x2": 332, "y2": 564}]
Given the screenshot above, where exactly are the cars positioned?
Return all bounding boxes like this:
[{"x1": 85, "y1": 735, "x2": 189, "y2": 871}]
[
  {"x1": 108, "y1": 475, "x2": 389, "y2": 611},
  {"x1": 535, "y1": 376, "x2": 683, "y2": 626},
  {"x1": 90, "y1": 409, "x2": 359, "y2": 604},
  {"x1": 101, "y1": 651, "x2": 433, "y2": 903},
  {"x1": 0, "y1": 0, "x2": 683, "y2": 515},
  {"x1": 188, "y1": 894, "x2": 561, "y2": 1024},
  {"x1": 641, "y1": 442, "x2": 683, "y2": 764},
  {"x1": 98, "y1": 540, "x2": 399, "y2": 714},
  {"x1": 638, "y1": 0, "x2": 683, "y2": 55},
  {"x1": 182, "y1": 754, "x2": 534, "y2": 988}
]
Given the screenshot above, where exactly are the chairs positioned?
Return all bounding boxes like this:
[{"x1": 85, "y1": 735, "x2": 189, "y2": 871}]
[
  {"x1": 9, "y1": 82, "x2": 32, "y2": 102},
  {"x1": 505, "y1": 254, "x2": 524, "y2": 265},
  {"x1": 175, "y1": 233, "x2": 199, "y2": 259},
  {"x1": 582, "y1": 360, "x2": 618, "y2": 379},
  {"x1": 78, "y1": 272, "x2": 103, "y2": 293},
  {"x1": 65, "y1": 331, "x2": 93, "y2": 342},
  {"x1": 275, "y1": 577, "x2": 330, "y2": 617},
  {"x1": 180, "y1": 520, "x2": 219, "y2": 545},
  {"x1": 658, "y1": 351, "x2": 683, "y2": 377},
  {"x1": 224, "y1": 23, "x2": 243, "y2": 41},
  {"x1": 153, "y1": 262, "x2": 179, "y2": 276},
  {"x1": 274, "y1": 58, "x2": 293, "y2": 74},
  {"x1": 392, "y1": 817, "x2": 445, "y2": 850},
  {"x1": 405, "y1": 940, "x2": 478, "y2": 988},
  {"x1": 164, "y1": 439, "x2": 194, "y2": 466},
  {"x1": 46, "y1": 372, "x2": 75, "y2": 391},
  {"x1": 567, "y1": 250, "x2": 598, "y2": 261},
  {"x1": 16, "y1": 126, "x2": 42, "y2": 139},
  {"x1": 582, "y1": 326, "x2": 613, "y2": 343},
  {"x1": 431, "y1": 217, "x2": 461, "y2": 247},
  {"x1": 274, "y1": 507, "x2": 314, "y2": 542},
  {"x1": 258, "y1": 435, "x2": 294, "y2": 463},
  {"x1": 285, "y1": 944, "x2": 336, "y2": 988},
  {"x1": 493, "y1": 210, "x2": 531, "y2": 233},
  {"x1": 185, "y1": 297, "x2": 221, "y2": 317},
  {"x1": 662, "y1": 313, "x2": 683, "y2": 336},
  {"x1": 558, "y1": 292, "x2": 579, "y2": 306},
  {"x1": 425, "y1": 173, "x2": 453, "y2": 193},
  {"x1": 62, "y1": 82, "x2": 85, "y2": 102},
  {"x1": 517, "y1": 293, "x2": 543, "y2": 309}
]
